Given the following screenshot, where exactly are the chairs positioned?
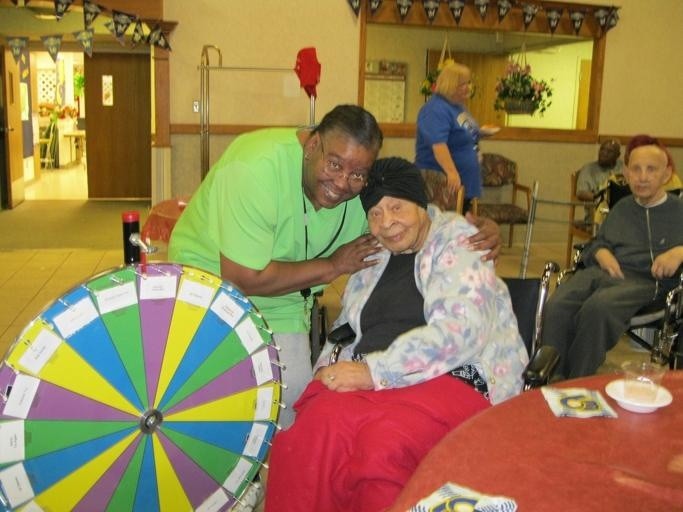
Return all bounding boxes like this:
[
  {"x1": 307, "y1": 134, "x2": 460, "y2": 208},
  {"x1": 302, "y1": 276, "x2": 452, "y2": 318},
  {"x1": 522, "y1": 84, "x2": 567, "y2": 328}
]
[
  {"x1": 421, "y1": 169, "x2": 465, "y2": 215},
  {"x1": 566, "y1": 168, "x2": 593, "y2": 268},
  {"x1": 471, "y1": 152, "x2": 531, "y2": 247}
]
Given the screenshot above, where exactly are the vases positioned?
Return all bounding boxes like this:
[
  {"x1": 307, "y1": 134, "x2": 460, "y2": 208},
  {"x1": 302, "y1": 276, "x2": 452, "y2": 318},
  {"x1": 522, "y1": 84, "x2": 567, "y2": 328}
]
[{"x1": 505, "y1": 96, "x2": 533, "y2": 114}]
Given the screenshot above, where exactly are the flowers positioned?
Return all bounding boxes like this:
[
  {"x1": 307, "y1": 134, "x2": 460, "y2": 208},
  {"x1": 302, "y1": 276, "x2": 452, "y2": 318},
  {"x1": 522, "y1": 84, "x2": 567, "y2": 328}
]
[{"x1": 493, "y1": 63, "x2": 555, "y2": 118}]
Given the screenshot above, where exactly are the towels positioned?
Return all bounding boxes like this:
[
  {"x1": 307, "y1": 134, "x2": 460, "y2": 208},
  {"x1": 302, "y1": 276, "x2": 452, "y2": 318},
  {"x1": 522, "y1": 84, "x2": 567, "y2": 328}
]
[
  {"x1": 540, "y1": 385, "x2": 618, "y2": 419},
  {"x1": 407, "y1": 481, "x2": 518, "y2": 512}
]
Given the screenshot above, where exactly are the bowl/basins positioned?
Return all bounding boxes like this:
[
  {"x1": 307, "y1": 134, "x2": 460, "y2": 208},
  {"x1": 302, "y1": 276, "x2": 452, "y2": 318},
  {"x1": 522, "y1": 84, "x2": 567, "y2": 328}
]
[{"x1": 604, "y1": 378, "x2": 673, "y2": 414}]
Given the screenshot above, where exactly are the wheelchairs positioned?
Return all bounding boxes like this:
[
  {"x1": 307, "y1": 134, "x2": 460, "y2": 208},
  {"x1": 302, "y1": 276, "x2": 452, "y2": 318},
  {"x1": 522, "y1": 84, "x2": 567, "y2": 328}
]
[
  {"x1": 327, "y1": 260, "x2": 565, "y2": 390},
  {"x1": 555, "y1": 181, "x2": 683, "y2": 372}
]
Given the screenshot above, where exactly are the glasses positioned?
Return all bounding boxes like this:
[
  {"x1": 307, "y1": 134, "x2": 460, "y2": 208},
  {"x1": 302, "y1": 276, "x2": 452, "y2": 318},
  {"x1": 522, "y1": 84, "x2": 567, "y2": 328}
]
[{"x1": 318, "y1": 135, "x2": 368, "y2": 183}]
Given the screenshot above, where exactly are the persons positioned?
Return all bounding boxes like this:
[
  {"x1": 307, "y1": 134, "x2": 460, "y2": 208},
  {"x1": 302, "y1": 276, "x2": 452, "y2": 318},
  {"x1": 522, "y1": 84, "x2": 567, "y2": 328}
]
[
  {"x1": 592, "y1": 133, "x2": 683, "y2": 222},
  {"x1": 163, "y1": 104, "x2": 503, "y2": 512},
  {"x1": 539, "y1": 146, "x2": 683, "y2": 383},
  {"x1": 572, "y1": 137, "x2": 624, "y2": 233},
  {"x1": 272, "y1": 158, "x2": 533, "y2": 512},
  {"x1": 413, "y1": 60, "x2": 481, "y2": 219}
]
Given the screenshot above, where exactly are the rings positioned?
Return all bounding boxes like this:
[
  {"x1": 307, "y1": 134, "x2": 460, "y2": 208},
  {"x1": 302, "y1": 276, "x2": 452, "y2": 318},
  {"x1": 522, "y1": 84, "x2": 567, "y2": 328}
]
[{"x1": 327, "y1": 374, "x2": 336, "y2": 381}]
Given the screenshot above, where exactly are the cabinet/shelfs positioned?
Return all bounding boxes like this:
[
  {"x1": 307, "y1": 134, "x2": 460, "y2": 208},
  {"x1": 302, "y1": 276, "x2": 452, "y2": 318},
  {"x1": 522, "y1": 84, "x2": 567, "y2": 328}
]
[
  {"x1": 197, "y1": 44, "x2": 321, "y2": 182},
  {"x1": 64, "y1": 129, "x2": 86, "y2": 162}
]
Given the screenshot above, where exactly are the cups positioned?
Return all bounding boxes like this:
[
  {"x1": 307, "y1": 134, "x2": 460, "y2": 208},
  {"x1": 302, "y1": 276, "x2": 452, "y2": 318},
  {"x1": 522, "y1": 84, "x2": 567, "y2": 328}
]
[{"x1": 621, "y1": 359, "x2": 670, "y2": 404}]
